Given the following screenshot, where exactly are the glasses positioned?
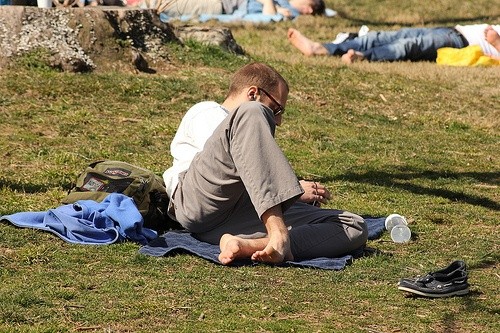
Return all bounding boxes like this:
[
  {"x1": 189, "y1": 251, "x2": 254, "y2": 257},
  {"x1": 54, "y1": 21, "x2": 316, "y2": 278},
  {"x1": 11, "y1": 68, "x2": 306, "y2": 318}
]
[{"x1": 257, "y1": 87, "x2": 285, "y2": 116}]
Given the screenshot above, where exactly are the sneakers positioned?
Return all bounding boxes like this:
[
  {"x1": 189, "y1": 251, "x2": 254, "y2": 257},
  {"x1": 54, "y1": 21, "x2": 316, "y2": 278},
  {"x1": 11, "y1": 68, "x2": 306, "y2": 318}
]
[{"x1": 398, "y1": 260, "x2": 470, "y2": 298}]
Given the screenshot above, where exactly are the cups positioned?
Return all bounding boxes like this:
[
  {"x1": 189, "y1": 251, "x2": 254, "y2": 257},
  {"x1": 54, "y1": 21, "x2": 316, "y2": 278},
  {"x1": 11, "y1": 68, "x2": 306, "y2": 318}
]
[{"x1": 385, "y1": 213, "x2": 411, "y2": 243}]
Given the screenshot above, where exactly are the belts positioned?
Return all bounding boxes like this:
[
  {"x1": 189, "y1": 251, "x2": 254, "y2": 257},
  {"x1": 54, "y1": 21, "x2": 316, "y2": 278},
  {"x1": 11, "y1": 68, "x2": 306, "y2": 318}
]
[{"x1": 449, "y1": 26, "x2": 469, "y2": 47}]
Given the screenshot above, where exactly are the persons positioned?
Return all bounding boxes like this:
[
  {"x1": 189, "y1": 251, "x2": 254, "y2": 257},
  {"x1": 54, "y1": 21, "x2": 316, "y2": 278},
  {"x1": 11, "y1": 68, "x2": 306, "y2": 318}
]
[
  {"x1": 162, "y1": 61, "x2": 369, "y2": 265},
  {"x1": 122, "y1": 0, "x2": 326, "y2": 17},
  {"x1": 285, "y1": 23, "x2": 500, "y2": 66}
]
